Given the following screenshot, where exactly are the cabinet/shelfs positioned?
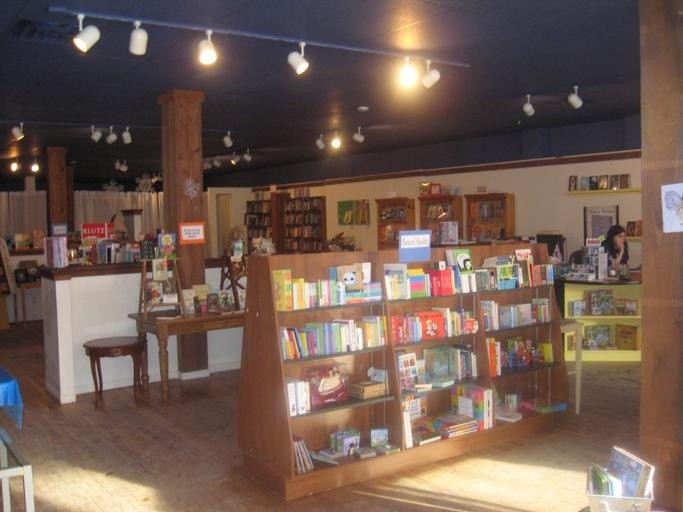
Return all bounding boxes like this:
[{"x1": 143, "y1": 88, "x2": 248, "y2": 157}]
[
  {"x1": 636, "y1": 0, "x2": 683, "y2": 511},
  {"x1": 551, "y1": 268, "x2": 643, "y2": 362},
  {"x1": 237, "y1": 242, "x2": 575, "y2": 502},
  {"x1": 463, "y1": 193, "x2": 515, "y2": 241},
  {"x1": 0, "y1": 366, "x2": 35, "y2": 511},
  {"x1": 374, "y1": 197, "x2": 415, "y2": 250},
  {"x1": 417, "y1": 195, "x2": 463, "y2": 244},
  {"x1": 566, "y1": 190, "x2": 643, "y2": 241},
  {"x1": 246, "y1": 192, "x2": 326, "y2": 254}
]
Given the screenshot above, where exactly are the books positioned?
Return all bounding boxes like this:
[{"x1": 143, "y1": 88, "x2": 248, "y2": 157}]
[
  {"x1": 626, "y1": 219, "x2": 642, "y2": 236},
  {"x1": 421, "y1": 198, "x2": 457, "y2": 242},
  {"x1": 273, "y1": 262, "x2": 389, "y2": 361},
  {"x1": 401, "y1": 382, "x2": 495, "y2": 448},
  {"x1": 571, "y1": 289, "x2": 642, "y2": 353},
  {"x1": 469, "y1": 201, "x2": 507, "y2": 240},
  {"x1": 482, "y1": 296, "x2": 554, "y2": 377},
  {"x1": 379, "y1": 206, "x2": 407, "y2": 241},
  {"x1": 246, "y1": 201, "x2": 272, "y2": 252},
  {"x1": 587, "y1": 447, "x2": 655, "y2": 499},
  {"x1": 568, "y1": 173, "x2": 631, "y2": 190},
  {"x1": 284, "y1": 199, "x2": 323, "y2": 253},
  {"x1": 390, "y1": 306, "x2": 478, "y2": 393},
  {"x1": 284, "y1": 376, "x2": 399, "y2": 474},
  {"x1": 384, "y1": 248, "x2": 553, "y2": 301}
]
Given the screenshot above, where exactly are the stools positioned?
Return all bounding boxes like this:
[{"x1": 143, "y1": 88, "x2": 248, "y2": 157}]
[{"x1": 84, "y1": 335, "x2": 148, "y2": 408}]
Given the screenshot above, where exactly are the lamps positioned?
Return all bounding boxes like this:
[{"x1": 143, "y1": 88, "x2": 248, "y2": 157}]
[
  {"x1": 287, "y1": 42, "x2": 310, "y2": 74},
  {"x1": 197, "y1": 29, "x2": 218, "y2": 66},
  {"x1": 422, "y1": 60, "x2": 441, "y2": 88},
  {"x1": 11, "y1": 86, "x2": 583, "y2": 184},
  {"x1": 127, "y1": 21, "x2": 149, "y2": 55},
  {"x1": 73, "y1": 14, "x2": 102, "y2": 54}
]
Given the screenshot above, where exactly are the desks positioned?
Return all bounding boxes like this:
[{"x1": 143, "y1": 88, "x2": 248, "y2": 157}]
[
  {"x1": 12, "y1": 282, "x2": 42, "y2": 330},
  {"x1": 127, "y1": 307, "x2": 243, "y2": 402}
]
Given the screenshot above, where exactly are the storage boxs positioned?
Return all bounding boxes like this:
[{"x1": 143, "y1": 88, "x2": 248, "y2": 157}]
[{"x1": 586, "y1": 468, "x2": 656, "y2": 512}]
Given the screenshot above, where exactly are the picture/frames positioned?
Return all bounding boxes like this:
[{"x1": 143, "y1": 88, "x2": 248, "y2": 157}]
[
  {"x1": 568, "y1": 174, "x2": 642, "y2": 236},
  {"x1": 419, "y1": 181, "x2": 441, "y2": 196}
]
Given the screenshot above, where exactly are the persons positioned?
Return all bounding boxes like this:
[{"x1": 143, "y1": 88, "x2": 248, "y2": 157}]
[
  {"x1": 601, "y1": 225, "x2": 629, "y2": 269},
  {"x1": 665, "y1": 191, "x2": 683, "y2": 226}
]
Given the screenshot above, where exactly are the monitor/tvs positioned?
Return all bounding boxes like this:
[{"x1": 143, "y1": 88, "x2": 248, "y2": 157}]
[{"x1": 536, "y1": 233, "x2": 564, "y2": 263}]
